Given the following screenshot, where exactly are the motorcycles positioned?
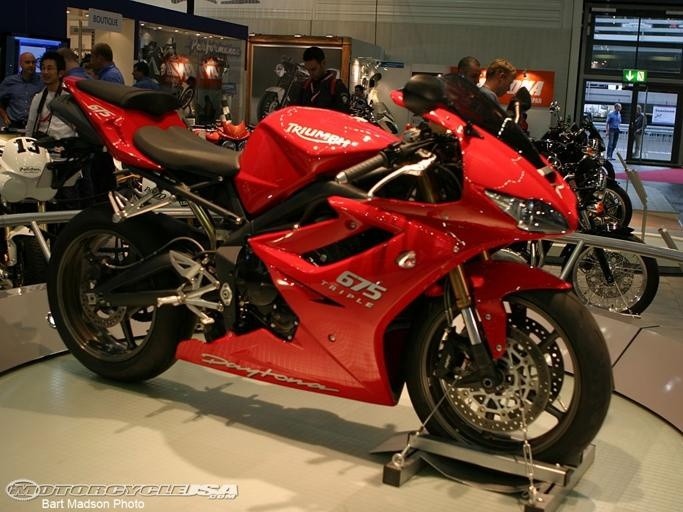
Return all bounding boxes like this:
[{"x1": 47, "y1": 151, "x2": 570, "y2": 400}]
[
  {"x1": 44, "y1": 67, "x2": 614, "y2": 485},
  {"x1": 258, "y1": 56, "x2": 310, "y2": 123},
  {"x1": 0, "y1": 51, "x2": 662, "y2": 336}
]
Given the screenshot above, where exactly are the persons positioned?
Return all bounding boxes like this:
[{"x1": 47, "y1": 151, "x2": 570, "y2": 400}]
[
  {"x1": 606, "y1": 103, "x2": 623, "y2": 160},
  {"x1": 160, "y1": 62, "x2": 167, "y2": 79},
  {"x1": 295, "y1": 47, "x2": 350, "y2": 115},
  {"x1": 0, "y1": 43, "x2": 124, "y2": 139},
  {"x1": 633, "y1": 104, "x2": 647, "y2": 156},
  {"x1": 478, "y1": 58, "x2": 517, "y2": 106},
  {"x1": 131, "y1": 61, "x2": 160, "y2": 89},
  {"x1": 457, "y1": 56, "x2": 480, "y2": 86},
  {"x1": 355, "y1": 85, "x2": 367, "y2": 109}
]
